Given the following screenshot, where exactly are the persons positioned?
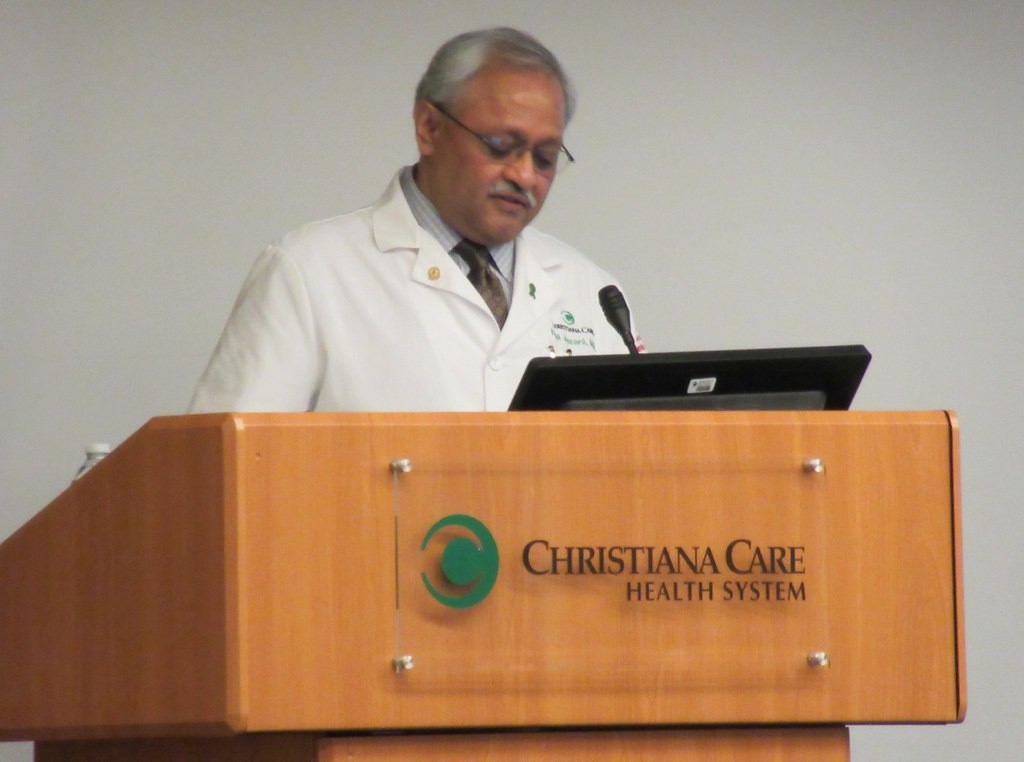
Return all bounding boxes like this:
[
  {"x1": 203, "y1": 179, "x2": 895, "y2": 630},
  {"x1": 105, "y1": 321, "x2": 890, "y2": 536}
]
[{"x1": 189, "y1": 26, "x2": 647, "y2": 415}]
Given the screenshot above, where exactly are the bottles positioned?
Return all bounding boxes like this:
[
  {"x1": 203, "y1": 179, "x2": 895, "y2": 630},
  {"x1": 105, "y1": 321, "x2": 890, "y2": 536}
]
[{"x1": 72, "y1": 444, "x2": 111, "y2": 483}]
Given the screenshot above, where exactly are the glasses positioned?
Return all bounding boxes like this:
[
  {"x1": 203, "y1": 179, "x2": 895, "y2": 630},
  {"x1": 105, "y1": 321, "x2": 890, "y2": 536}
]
[{"x1": 434, "y1": 103, "x2": 575, "y2": 177}]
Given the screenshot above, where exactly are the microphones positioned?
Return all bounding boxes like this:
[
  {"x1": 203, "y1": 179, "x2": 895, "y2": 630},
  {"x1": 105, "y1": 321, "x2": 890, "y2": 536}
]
[{"x1": 598, "y1": 285, "x2": 638, "y2": 354}]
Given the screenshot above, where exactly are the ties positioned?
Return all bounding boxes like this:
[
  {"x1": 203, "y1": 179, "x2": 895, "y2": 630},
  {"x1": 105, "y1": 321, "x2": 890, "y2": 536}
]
[{"x1": 454, "y1": 239, "x2": 508, "y2": 332}]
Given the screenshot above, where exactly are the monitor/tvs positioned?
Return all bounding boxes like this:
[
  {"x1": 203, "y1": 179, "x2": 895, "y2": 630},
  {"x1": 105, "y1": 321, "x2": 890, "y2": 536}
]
[{"x1": 508, "y1": 342, "x2": 872, "y2": 414}]
